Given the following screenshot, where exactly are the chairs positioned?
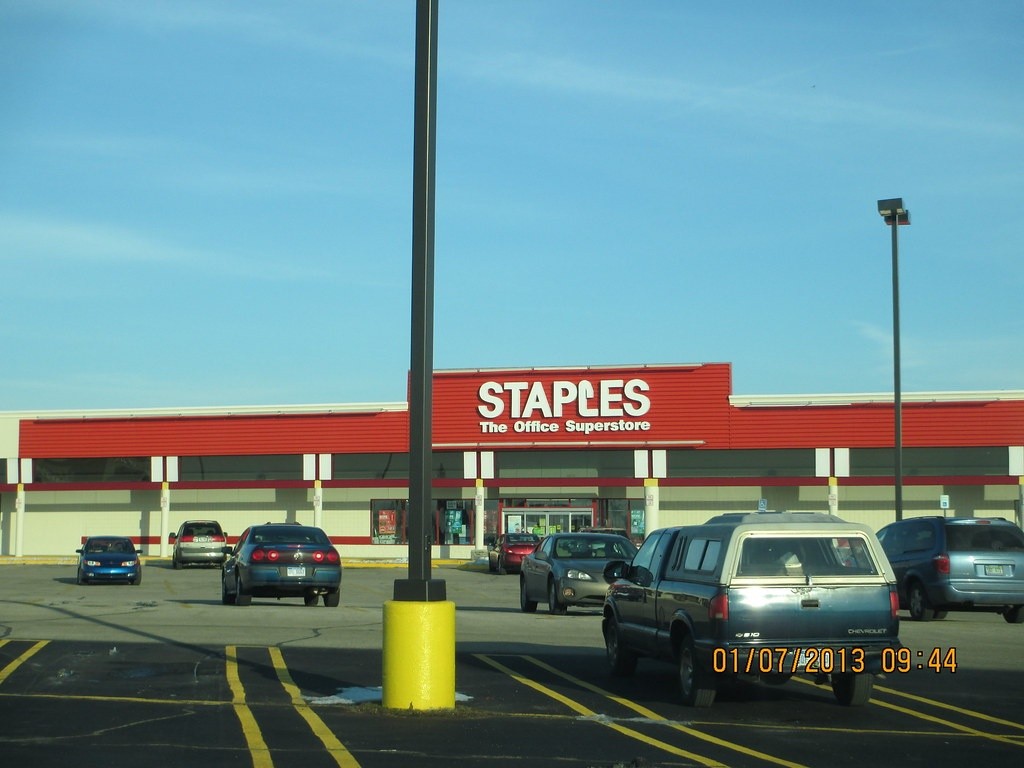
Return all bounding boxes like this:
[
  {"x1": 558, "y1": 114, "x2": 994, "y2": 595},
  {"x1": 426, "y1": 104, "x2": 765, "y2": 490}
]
[
  {"x1": 110, "y1": 541, "x2": 126, "y2": 552},
  {"x1": 208, "y1": 530, "x2": 214, "y2": 535},
  {"x1": 93, "y1": 543, "x2": 101, "y2": 551},
  {"x1": 187, "y1": 528, "x2": 194, "y2": 536}
]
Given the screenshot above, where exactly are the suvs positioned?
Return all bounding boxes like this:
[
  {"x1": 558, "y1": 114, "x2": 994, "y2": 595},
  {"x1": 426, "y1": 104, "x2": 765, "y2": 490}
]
[
  {"x1": 603, "y1": 511, "x2": 899, "y2": 708},
  {"x1": 876, "y1": 516, "x2": 1023, "y2": 622}
]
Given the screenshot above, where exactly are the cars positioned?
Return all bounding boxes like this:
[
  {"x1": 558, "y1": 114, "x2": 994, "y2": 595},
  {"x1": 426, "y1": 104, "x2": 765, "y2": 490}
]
[
  {"x1": 489, "y1": 533, "x2": 540, "y2": 574},
  {"x1": 76, "y1": 536, "x2": 143, "y2": 585},
  {"x1": 221, "y1": 522, "x2": 342, "y2": 607},
  {"x1": 169, "y1": 521, "x2": 228, "y2": 570},
  {"x1": 520, "y1": 532, "x2": 639, "y2": 615}
]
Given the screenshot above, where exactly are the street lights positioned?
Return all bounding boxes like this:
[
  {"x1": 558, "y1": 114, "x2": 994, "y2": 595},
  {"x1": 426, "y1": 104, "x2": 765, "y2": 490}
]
[{"x1": 876, "y1": 199, "x2": 910, "y2": 519}]
